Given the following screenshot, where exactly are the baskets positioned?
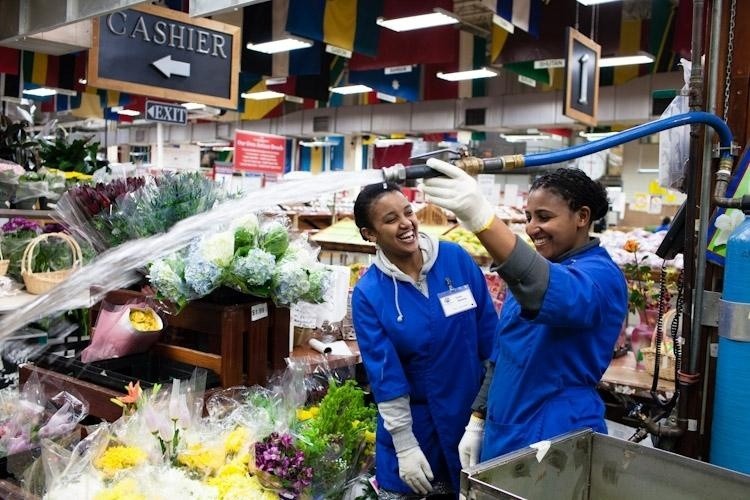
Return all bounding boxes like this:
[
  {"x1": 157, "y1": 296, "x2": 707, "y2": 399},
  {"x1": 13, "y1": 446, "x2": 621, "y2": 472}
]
[{"x1": 0, "y1": 259, "x2": 90, "y2": 299}]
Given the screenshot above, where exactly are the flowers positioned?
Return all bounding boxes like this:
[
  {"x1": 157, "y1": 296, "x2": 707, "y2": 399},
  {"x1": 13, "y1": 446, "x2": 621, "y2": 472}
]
[
  {"x1": 149, "y1": 217, "x2": 369, "y2": 311},
  {"x1": 642, "y1": 267, "x2": 680, "y2": 310},
  {"x1": 81, "y1": 303, "x2": 163, "y2": 364},
  {"x1": 620, "y1": 239, "x2": 659, "y2": 323},
  {"x1": 1, "y1": 378, "x2": 378, "y2": 500},
  {"x1": 1, "y1": 160, "x2": 219, "y2": 272}
]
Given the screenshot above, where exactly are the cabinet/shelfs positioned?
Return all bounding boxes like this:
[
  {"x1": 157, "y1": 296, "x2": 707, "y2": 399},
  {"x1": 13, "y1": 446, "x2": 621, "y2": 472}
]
[
  {"x1": 460, "y1": 227, "x2": 494, "y2": 269},
  {"x1": 402, "y1": 203, "x2": 460, "y2": 242},
  {"x1": 259, "y1": 191, "x2": 377, "y2": 269},
  {"x1": 586, "y1": 169, "x2": 621, "y2": 270},
  {"x1": 475, "y1": 169, "x2": 537, "y2": 250}
]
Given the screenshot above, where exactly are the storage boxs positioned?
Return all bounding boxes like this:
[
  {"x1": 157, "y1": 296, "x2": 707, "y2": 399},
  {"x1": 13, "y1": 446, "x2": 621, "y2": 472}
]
[{"x1": 460, "y1": 426, "x2": 750, "y2": 500}]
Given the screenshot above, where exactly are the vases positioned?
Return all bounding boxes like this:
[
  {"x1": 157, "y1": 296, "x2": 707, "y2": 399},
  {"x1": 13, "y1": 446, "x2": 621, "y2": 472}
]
[
  {"x1": 631, "y1": 322, "x2": 654, "y2": 370},
  {"x1": 645, "y1": 306, "x2": 661, "y2": 331}
]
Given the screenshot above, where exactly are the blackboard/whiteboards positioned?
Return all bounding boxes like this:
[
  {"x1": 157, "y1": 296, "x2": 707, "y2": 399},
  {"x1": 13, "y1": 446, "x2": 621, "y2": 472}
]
[
  {"x1": 86, "y1": 3, "x2": 242, "y2": 110},
  {"x1": 562, "y1": 26, "x2": 602, "y2": 127}
]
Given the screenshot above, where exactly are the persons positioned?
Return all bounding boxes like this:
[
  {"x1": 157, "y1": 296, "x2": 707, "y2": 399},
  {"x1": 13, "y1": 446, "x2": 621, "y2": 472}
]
[
  {"x1": 420, "y1": 153, "x2": 631, "y2": 473},
  {"x1": 350, "y1": 182, "x2": 500, "y2": 500}
]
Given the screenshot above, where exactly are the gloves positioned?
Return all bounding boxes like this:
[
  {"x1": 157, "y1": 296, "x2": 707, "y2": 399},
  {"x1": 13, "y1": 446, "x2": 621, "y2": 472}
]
[
  {"x1": 458, "y1": 414, "x2": 486, "y2": 470},
  {"x1": 396, "y1": 444, "x2": 434, "y2": 495},
  {"x1": 423, "y1": 157, "x2": 496, "y2": 235}
]
